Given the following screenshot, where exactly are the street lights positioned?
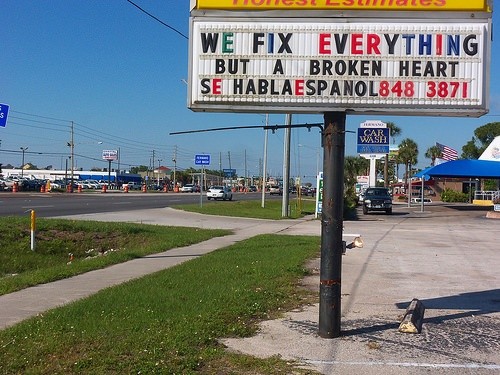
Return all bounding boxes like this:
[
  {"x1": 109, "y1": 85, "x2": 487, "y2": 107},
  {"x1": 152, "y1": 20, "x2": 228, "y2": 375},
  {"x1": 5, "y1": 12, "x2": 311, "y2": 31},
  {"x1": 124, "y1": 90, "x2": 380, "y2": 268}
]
[
  {"x1": 70, "y1": 141, "x2": 104, "y2": 193},
  {"x1": 298, "y1": 143, "x2": 321, "y2": 175},
  {"x1": 157, "y1": 159, "x2": 163, "y2": 185},
  {"x1": 20, "y1": 146, "x2": 28, "y2": 177}
]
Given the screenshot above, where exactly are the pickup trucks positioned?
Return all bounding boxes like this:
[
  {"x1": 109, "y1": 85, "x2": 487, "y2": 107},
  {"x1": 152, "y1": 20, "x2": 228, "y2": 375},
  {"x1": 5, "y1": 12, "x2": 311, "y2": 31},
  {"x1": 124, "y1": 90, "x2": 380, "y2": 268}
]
[{"x1": 122, "y1": 182, "x2": 139, "y2": 190}]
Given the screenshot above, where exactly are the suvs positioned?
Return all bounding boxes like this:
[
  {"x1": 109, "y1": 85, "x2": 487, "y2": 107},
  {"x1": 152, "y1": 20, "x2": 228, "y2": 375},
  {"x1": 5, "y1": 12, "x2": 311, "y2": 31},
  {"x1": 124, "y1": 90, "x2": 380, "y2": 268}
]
[{"x1": 360, "y1": 186, "x2": 393, "y2": 215}]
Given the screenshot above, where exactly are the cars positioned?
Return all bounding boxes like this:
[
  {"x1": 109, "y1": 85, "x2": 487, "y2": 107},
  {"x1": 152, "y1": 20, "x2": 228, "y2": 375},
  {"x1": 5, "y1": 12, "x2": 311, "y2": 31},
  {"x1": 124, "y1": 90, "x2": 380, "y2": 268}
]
[
  {"x1": 0, "y1": 175, "x2": 118, "y2": 191},
  {"x1": 289, "y1": 185, "x2": 316, "y2": 198},
  {"x1": 269, "y1": 185, "x2": 281, "y2": 196},
  {"x1": 146, "y1": 184, "x2": 164, "y2": 191},
  {"x1": 206, "y1": 186, "x2": 233, "y2": 201},
  {"x1": 181, "y1": 184, "x2": 197, "y2": 193}
]
